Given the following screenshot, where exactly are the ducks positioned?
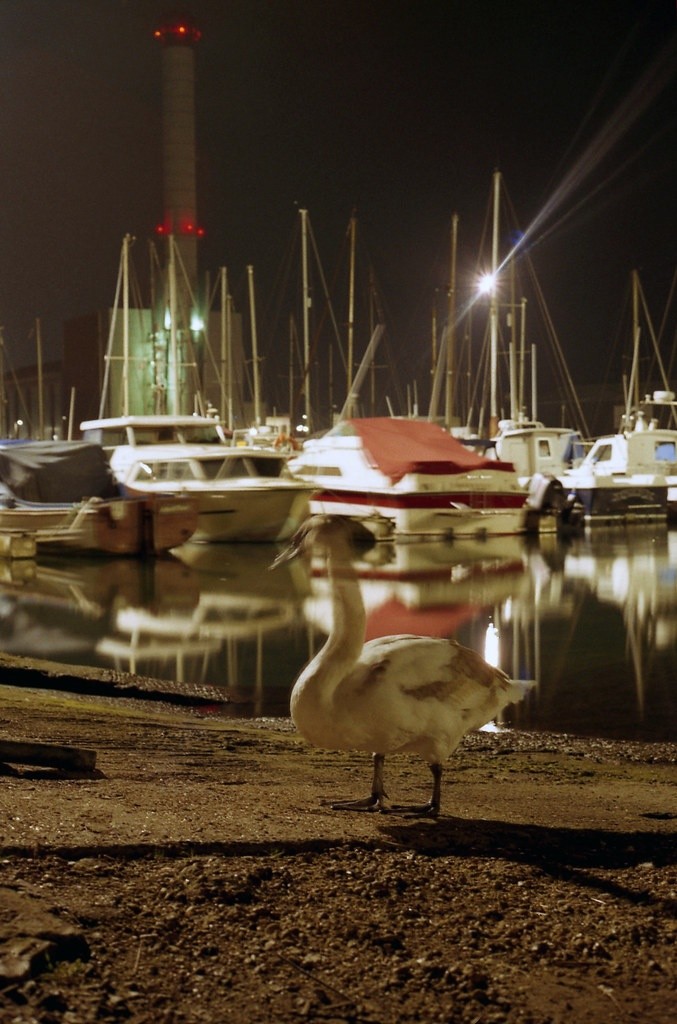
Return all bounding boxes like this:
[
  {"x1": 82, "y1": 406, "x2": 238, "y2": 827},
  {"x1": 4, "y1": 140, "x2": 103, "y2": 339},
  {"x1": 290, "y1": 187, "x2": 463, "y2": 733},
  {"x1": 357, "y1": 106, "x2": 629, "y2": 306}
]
[{"x1": 266, "y1": 513, "x2": 539, "y2": 818}]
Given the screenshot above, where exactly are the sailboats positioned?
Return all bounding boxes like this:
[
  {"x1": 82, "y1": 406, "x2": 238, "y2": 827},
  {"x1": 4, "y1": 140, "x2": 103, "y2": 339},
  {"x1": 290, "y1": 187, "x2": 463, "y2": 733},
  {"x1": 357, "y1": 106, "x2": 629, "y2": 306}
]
[{"x1": 0, "y1": 170, "x2": 677, "y2": 558}]
